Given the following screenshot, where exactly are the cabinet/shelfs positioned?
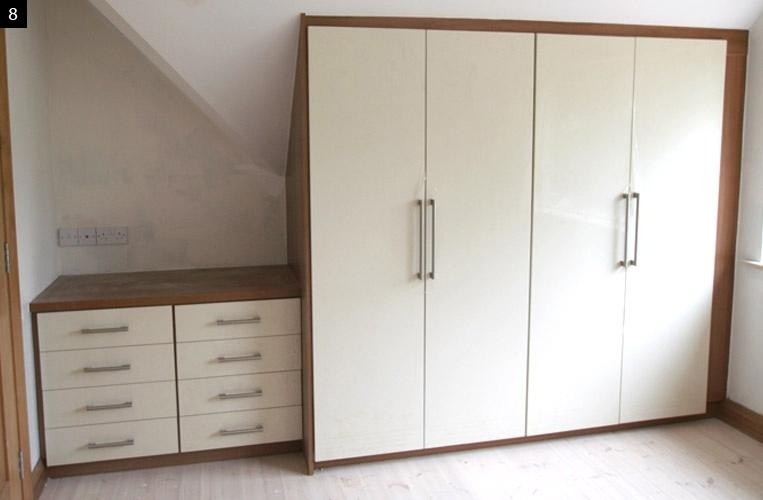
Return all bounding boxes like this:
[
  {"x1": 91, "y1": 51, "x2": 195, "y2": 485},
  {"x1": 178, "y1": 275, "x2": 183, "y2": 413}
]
[
  {"x1": 286, "y1": 13, "x2": 748, "y2": 474},
  {"x1": 28, "y1": 263, "x2": 304, "y2": 478}
]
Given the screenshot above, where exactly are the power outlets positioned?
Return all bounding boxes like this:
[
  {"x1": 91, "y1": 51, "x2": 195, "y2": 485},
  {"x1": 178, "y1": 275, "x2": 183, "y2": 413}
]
[{"x1": 96, "y1": 226, "x2": 127, "y2": 245}]
